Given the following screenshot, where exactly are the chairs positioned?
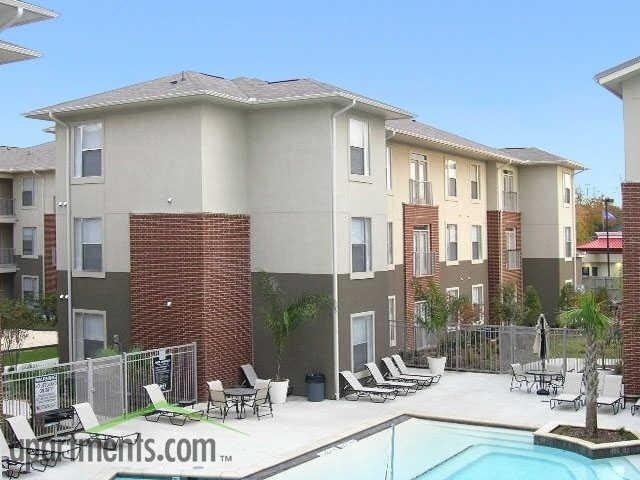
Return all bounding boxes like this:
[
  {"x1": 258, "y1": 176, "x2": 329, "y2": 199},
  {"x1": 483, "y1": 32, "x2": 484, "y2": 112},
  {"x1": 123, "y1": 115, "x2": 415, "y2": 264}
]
[
  {"x1": 140, "y1": 383, "x2": 204, "y2": 426},
  {"x1": 0, "y1": 431, "x2": 49, "y2": 480},
  {"x1": 69, "y1": 402, "x2": 140, "y2": 450},
  {"x1": 3, "y1": 415, "x2": 81, "y2": 467},
  {"x1": 206, "y1": 379, "x2": 239, "y2": 423},
  {"x1": 242, "y1": 378, "x2": 273, "y2": 420},
  {"x1": 339, "y1": 354, "x2": 441, "y2": 403},
  {"x1": 550, "y1": 363, "x2": 640, "y2": 416},
  {"x1": 239, "y1": 363, "x2": 258, "y2": 388},
  {"x1": 510, "y1": 363, "x2": 532, "y2": 393}
]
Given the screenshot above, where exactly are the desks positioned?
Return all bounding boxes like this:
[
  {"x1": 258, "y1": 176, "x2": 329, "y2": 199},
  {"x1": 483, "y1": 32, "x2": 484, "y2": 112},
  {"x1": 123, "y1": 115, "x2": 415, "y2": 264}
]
[
  {"x1": 525, "y1": 370, "x2": 560, "y2": 397},
  {"x1": 222, "y1": 389, "x2": 257, "y2": 419}
]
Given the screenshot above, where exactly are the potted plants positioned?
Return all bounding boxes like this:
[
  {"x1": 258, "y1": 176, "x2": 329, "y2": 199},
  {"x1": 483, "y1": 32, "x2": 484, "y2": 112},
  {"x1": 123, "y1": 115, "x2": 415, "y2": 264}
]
[
  {"x1": 259, "y1": 271, "x2": 321, "y2": 400},
  {"x1": 406, "y1": 276, "x2": 468, "y2": 376}
]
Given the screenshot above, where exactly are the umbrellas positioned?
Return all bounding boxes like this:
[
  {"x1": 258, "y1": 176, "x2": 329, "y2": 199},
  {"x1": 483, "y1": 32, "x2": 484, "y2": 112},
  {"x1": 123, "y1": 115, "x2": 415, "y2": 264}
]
[{"x1": 533, "y1": 313, "x2": 551, "y2": 389}]
